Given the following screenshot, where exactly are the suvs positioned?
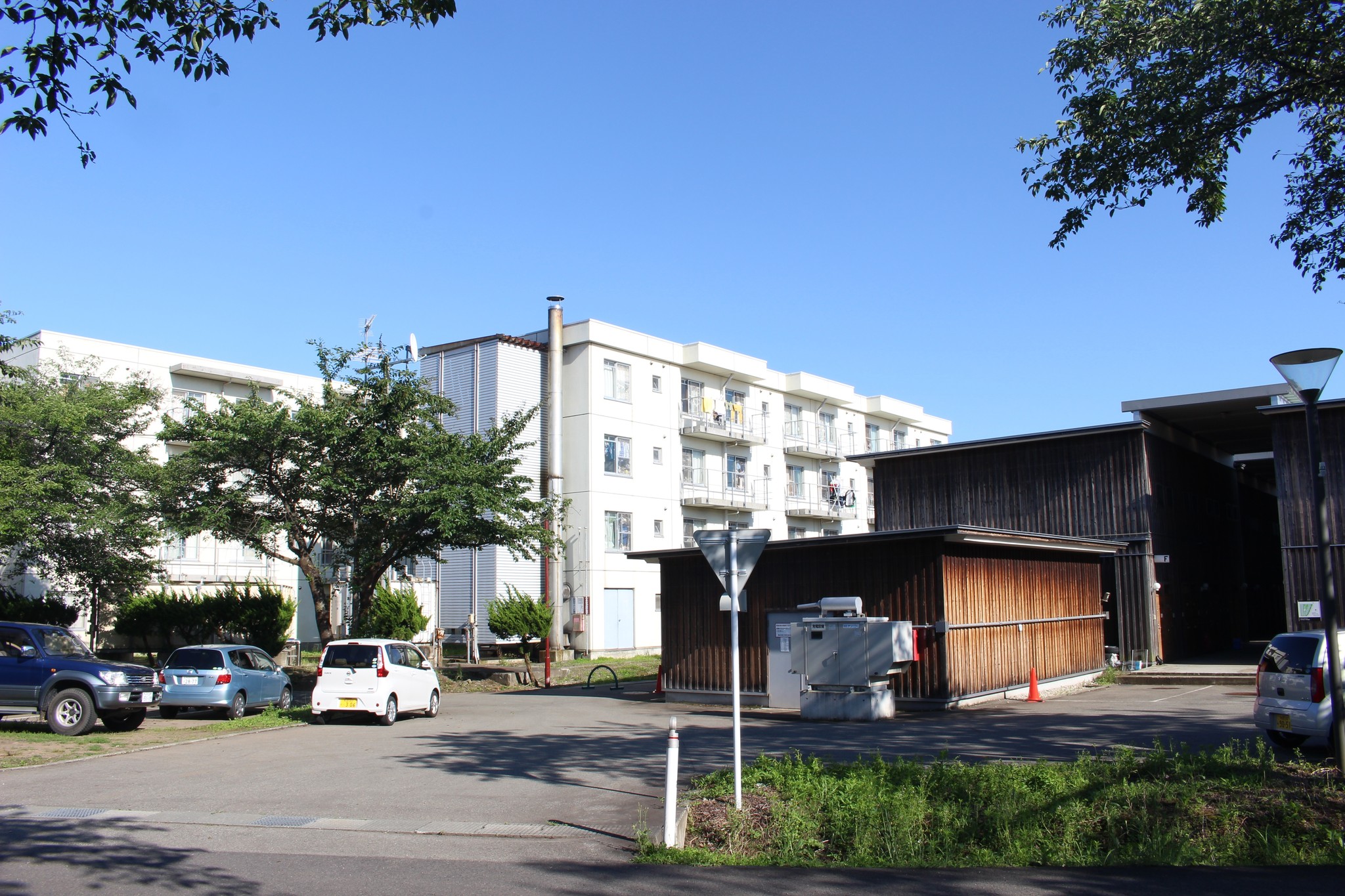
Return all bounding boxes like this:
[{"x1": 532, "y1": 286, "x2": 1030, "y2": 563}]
[{"x1": 0, "y1": 620, "x2": 165, "y2": 738}]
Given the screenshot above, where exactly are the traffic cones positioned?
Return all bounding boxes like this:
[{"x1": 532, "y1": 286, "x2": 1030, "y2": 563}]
[
  {"x1": 652, "y1": 665, "x2": 664, "y2": 694},
  {"x1": 1026, "y1": 667, "x2": 1044, "y2": 703}
]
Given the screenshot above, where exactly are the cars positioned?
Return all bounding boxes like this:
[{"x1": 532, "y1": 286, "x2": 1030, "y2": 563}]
[
  {"x1": 158, "y1": 644, "x2": 294, "y2": 720},
  {"x1": 310, "y1": 638, "x2": 441, "y2": 727},
  {"x1": 1253, "y1": 627, "x2": 1345, "y2": 753}
]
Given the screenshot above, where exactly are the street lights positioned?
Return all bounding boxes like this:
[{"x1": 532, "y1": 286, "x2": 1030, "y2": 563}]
[{"x1": 1270, "y1": 348, "x2": 1345, "y2": 778}]
